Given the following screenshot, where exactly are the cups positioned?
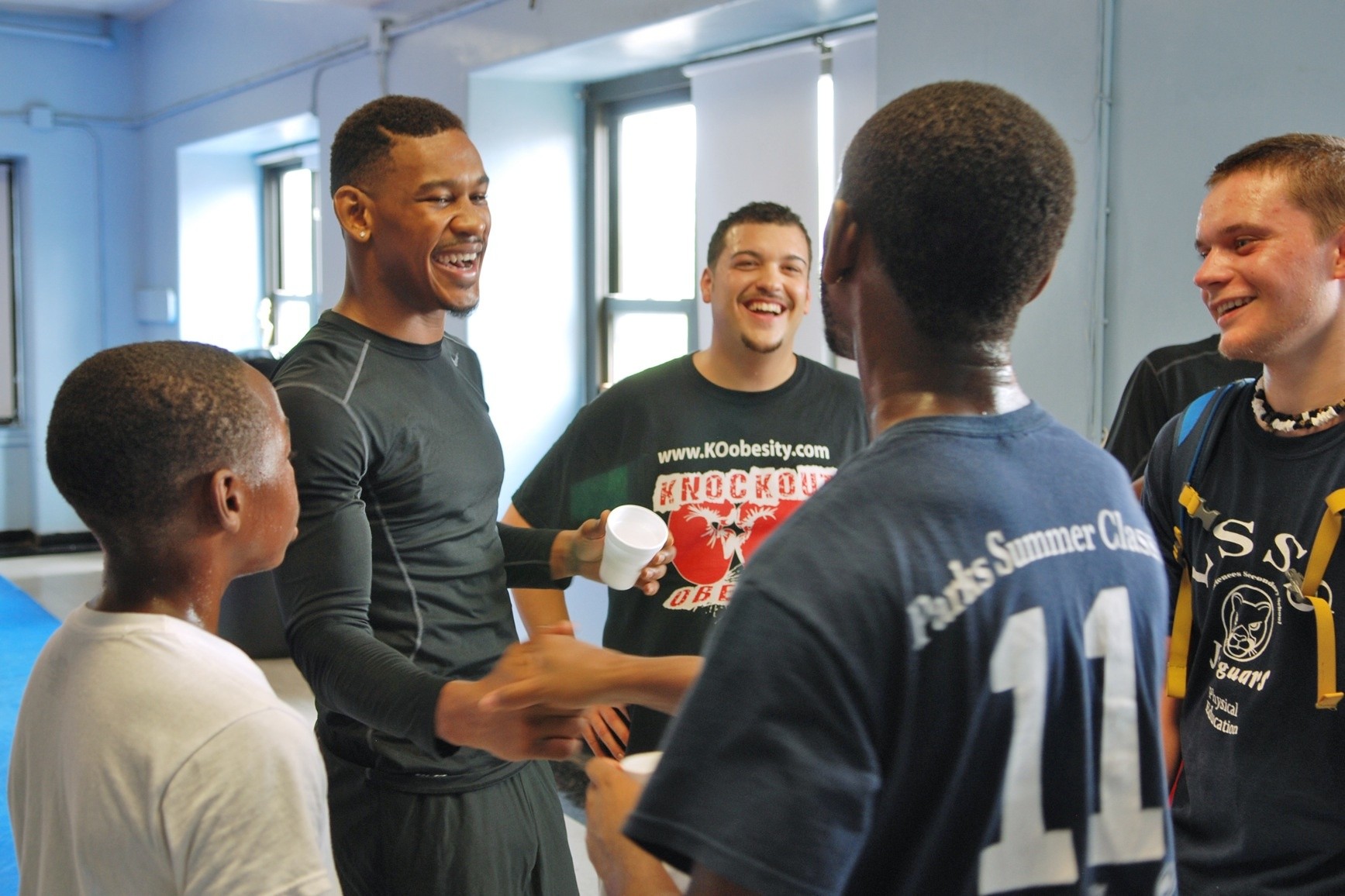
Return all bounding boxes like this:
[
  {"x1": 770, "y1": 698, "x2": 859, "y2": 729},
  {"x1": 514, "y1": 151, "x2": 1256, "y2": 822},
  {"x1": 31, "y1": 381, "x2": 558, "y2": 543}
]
[
  {"x1": 620, "y1": 751, "x2": 664, "y2": 787},
  {"x1": 600, "y1": 504, "x2": 668, "y2": 591}
]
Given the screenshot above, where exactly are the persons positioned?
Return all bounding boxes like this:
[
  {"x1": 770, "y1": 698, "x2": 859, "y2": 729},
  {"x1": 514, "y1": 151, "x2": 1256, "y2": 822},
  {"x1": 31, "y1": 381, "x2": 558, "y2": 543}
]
[
  {"x1": 1102, "y1": 332, "x2": 1268, "y2": 502},
  {"x1": 8, "y1": 341, "x2": 346, "y2": 895},
  {"x1": 260, "y1": 95, "x2": 676, "y2": 895},
  {"x1": 1141, "y1": 131, "x2": 1345, "y2": 896},
  {"x1": 502, "y1": 204, "x2": 869, "y2": 764},
  {"x1": 585, "y1": 81, "x2": 1189, "y2": 896}
]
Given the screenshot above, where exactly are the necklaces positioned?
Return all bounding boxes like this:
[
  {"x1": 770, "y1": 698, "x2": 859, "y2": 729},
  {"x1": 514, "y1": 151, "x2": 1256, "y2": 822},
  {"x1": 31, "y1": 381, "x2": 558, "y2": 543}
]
[{"x1": 1252, "y1": 375, "x2": 1345, "y2": 431}]
